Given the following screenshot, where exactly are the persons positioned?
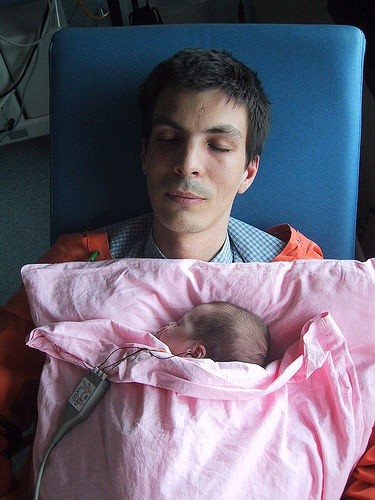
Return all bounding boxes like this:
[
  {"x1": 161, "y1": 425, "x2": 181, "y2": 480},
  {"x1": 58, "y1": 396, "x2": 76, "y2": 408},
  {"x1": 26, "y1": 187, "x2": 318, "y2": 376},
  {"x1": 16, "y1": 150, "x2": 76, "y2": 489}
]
[
  {"x1": 152, "y1": 301, "x2": 271, "y2": 369},
  {"x1": 0, "y1": 47, "x2": 375, "y2": 500}
]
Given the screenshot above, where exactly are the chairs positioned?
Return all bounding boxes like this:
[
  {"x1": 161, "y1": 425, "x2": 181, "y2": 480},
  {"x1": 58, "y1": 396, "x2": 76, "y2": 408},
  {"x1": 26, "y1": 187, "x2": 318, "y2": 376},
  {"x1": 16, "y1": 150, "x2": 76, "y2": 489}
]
[{"x1": 49, "y1": 23, "x2": 367, "y2": 277}]
